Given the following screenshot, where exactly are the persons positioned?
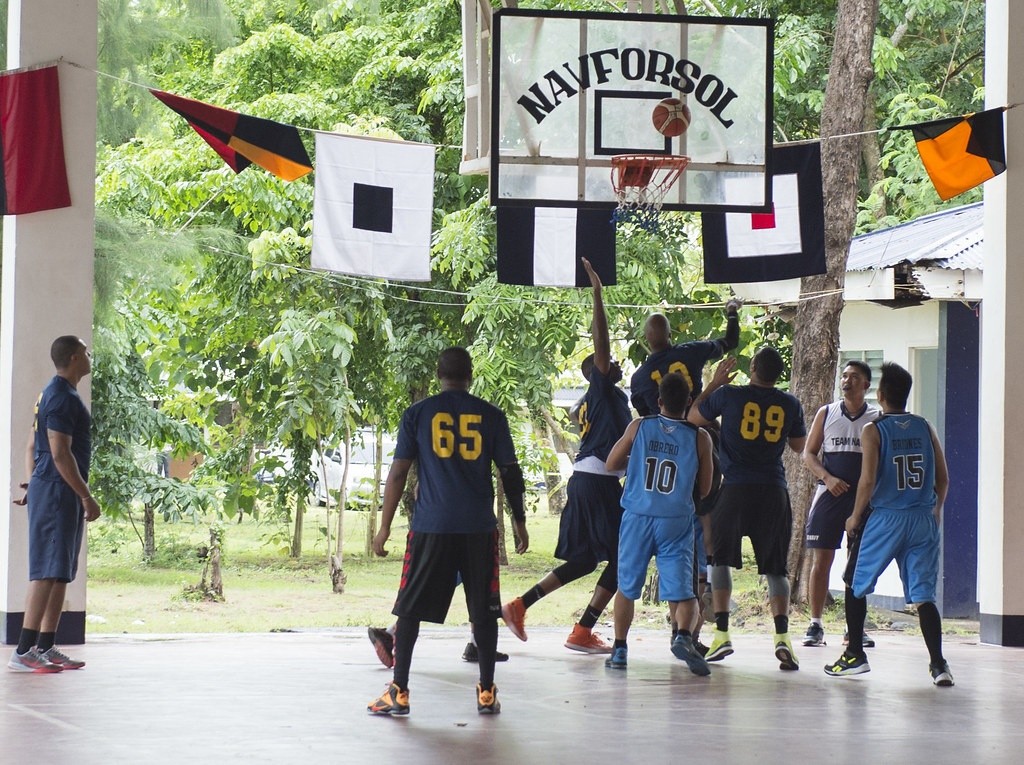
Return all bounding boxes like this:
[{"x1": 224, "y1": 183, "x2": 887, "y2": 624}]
[
  {"x1": 628, "y1": 297, "x2": 746, "y2": 622},
  {"x1": 501, "y1": 254, "x2": 633, "y2": 654},
  {"x1": 687, "y1": 346, "x2": 807, "y2": 672},
  {"x1": 7, "y1": 333, "x2": 105, "y2": 674},
  {"x1": 367, "y1": 348, "x2": 529, "y2": 714},
  {"x1": 368, "y1": 482, "x2": 510, "y2": 671},
  {"x1": 823, "y1": 361, "x2": 960, "y2": 688},
  {"x1": 799, "y1": 358, "x2": 883, "y2": 647},
  {"x1": 603, "y1": 372, "x2": 717, "y2": 676},
  {"x1": 155, "y1": 445, "x2": 172, "y2": 477}
]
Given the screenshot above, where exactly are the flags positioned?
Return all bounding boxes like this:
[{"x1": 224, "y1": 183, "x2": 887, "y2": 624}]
[
  {"x1": 310, "y1": 131, "x2": 433, "y2": 281},
  {"x1": 496, "y1": 175, "x2": 616, "y2": 289},
  {"x1": 149, "y1": 87, "x2": 314, "y2": 187},
  {"x1": 0, "y1": 58, "x2": 73, "y2": 217},
  {"x1": 700, "y1": 142, "x2": 829, "y2": 285},
  {"x1": 910, "y1": 109, "x2": 1007, "y2": 201}
]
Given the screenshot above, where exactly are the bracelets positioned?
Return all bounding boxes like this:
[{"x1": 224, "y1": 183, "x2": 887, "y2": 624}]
[{"x1": 82, "y1": 494, "x2": 94, "y2": 502}]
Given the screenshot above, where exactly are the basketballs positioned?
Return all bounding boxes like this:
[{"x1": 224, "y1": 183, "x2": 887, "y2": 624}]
[{"x1": 653, "y1": 98, "x2": 691, "y2": 138}]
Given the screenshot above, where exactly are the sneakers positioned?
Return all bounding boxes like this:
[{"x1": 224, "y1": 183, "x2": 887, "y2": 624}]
[
  {"x1": 775, "y1": 633, "x2": 799, "y2": 670},
  {"x1": 463, "y1": 642, "x2": 509, "y2": 663},
  {"x1": 701, "y1": 583, "x2": 717, "y2": 623},
  {"x1": 694, "y1": 641, "x2": 725, "y2": 661},
  {"x1": 368, "y1": 679, "x2": 410, "y2": 715},
  {"x1": 604, "y1": 645, "x2": 627, "y2": 670},
  {"x1": 702, "y1": 628, "x2": 734, "y2": 660},
  {"x1": 476, "y1": 681, "x2": 501, "y2": 715},
  {"x1": 824, "y1": 649, "x2": 871, "y2": 676},
  {"x1": 565, "y1": 625, "x2": 613, "y2": 655},
  {"x1": 802, "y1": 622, "x2": 825, "y2": 646},
  {"x1": 502, "y1": 596, "x2": 529, "y2": 643},
  {"x1": 367, "y1": 626, "x2": 394, "y2": 669},
  {"x1": 671, "y1": 633, "x2": 710, "y2": 676},
  {"x1": 929, "y1": 659, "x2": 955, "y2": 687},
  {"x1": 37, "y1": 644, "x2": 86, "y2": 671},
  {"x1": 8, "y1": 644, "x2": 63, "y2": 672},
  {"x1": 842, "y1": 627, "x2": 875, "y2": 648}
]
